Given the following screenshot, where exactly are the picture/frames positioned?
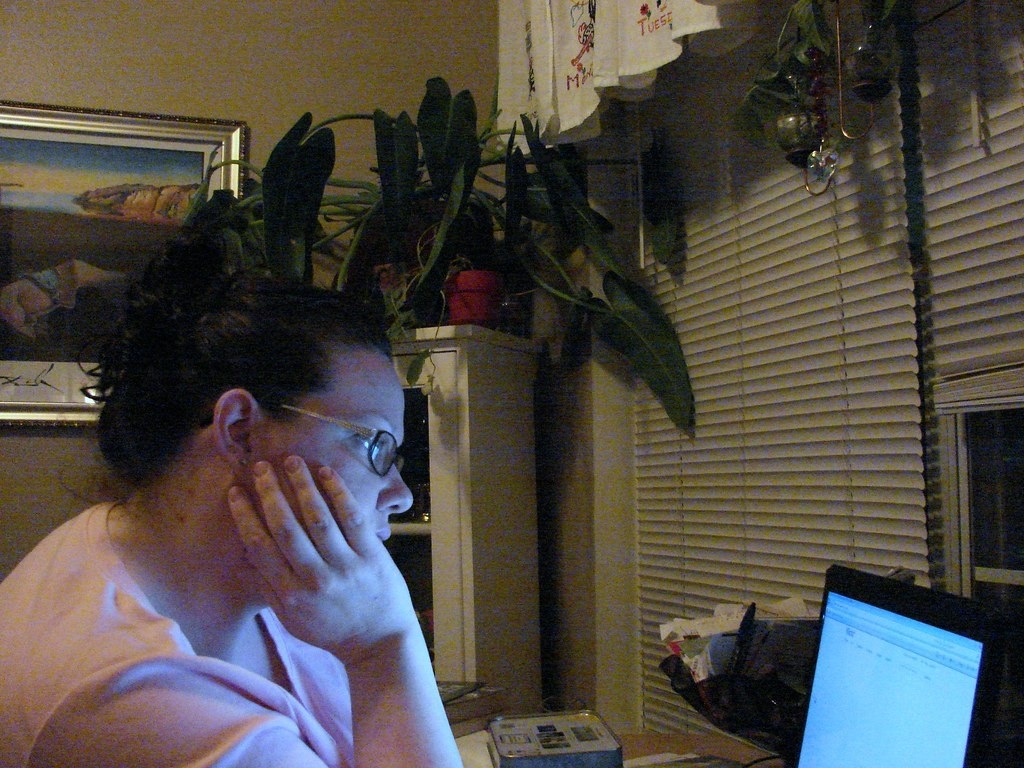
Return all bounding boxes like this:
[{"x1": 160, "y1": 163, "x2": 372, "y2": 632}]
[{"x1": 0, "y1": 100, "x2": 242, "y2": 429}]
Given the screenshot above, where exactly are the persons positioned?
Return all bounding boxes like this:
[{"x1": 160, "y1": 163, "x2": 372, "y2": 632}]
[{"x1": 0, "y1": 224, "x2": 463, "y2": 768}]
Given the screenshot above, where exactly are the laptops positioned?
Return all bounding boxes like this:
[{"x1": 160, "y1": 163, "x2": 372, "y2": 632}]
[{"x1": 781, "y1": 564, "x2": 1005, "y2": 768}]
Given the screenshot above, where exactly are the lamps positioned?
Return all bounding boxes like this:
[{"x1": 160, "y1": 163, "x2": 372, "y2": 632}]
[
  {"x1": 773, "y1": 48, "x2": 836, "y2": 195},
  {"x1": 848, "y1": 0, "x2": 893, "y2": 100}
]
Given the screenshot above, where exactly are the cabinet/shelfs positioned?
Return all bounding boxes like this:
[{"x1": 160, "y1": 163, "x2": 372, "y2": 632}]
[{"x1": 390, "y1": 325, "x2": 540, "y2": 710}]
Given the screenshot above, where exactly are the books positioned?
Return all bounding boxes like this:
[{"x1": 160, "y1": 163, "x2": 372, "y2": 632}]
[{"x1": 437, "y1": 681, "x2": 512, "y2": 739}]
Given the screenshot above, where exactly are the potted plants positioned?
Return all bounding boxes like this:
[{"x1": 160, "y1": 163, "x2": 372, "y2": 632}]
[{"x1": 177, "y1": 77, "x2": 697, "y2": 435}]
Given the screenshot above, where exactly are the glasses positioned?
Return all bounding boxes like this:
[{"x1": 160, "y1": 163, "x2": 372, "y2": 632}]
[{"x1": 198, "y1": 400, "x2": 409, "y2": 477}]
